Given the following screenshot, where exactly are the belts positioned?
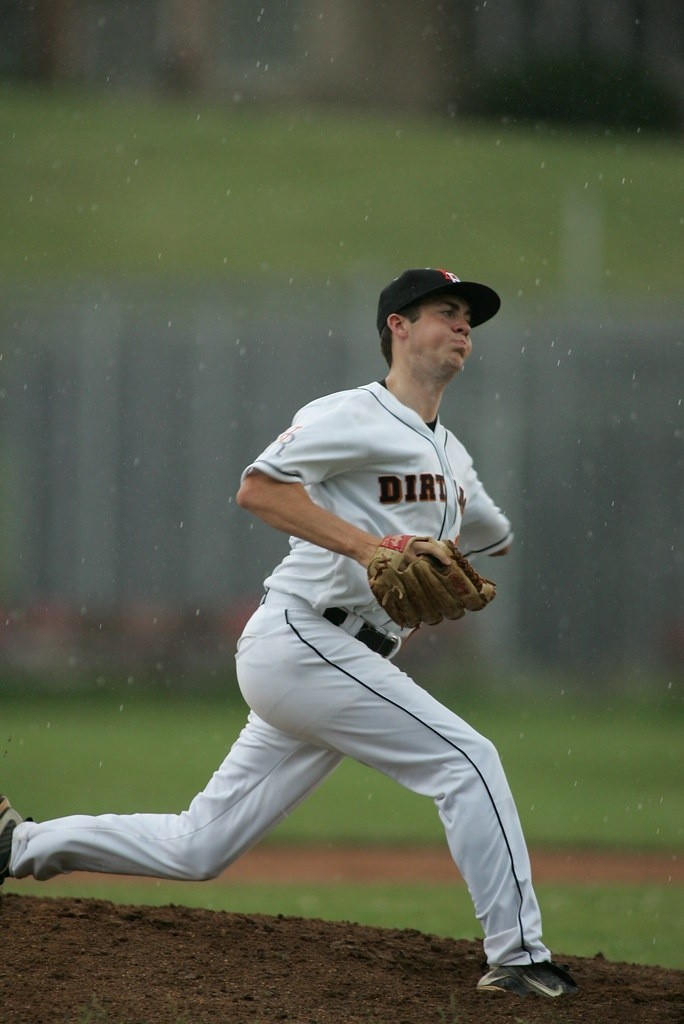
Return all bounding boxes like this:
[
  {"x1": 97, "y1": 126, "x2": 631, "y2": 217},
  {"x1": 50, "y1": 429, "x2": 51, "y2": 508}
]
[{"x1": 321, "y1": 606, "x2": 401, "y2": 659}]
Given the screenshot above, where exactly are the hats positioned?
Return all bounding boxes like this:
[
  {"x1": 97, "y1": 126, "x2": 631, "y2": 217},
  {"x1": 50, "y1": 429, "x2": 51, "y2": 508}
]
[{"x1": 377, "y1": 268, "x2": 501, "y2": 339}]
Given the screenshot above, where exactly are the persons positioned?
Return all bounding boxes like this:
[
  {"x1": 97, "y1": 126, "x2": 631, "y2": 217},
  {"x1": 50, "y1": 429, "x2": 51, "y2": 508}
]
[{"x1": 0, "y1": 268, "x2": 586, "y2": 1004}]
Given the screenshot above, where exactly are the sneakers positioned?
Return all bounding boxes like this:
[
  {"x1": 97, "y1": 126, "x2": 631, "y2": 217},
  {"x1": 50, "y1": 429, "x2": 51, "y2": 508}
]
[
  {"x1": 0, "y1": 796, "x2": 33, "y2": 886},
  {"x1": 476, "y1": 961, "x2": 581, "y2": 999}
]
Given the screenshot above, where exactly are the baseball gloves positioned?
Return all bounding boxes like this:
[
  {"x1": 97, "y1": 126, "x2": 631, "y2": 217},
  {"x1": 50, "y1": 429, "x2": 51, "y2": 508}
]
[{"x1": 368, "y1": 532, "x2": 498, "y2": 631}]
